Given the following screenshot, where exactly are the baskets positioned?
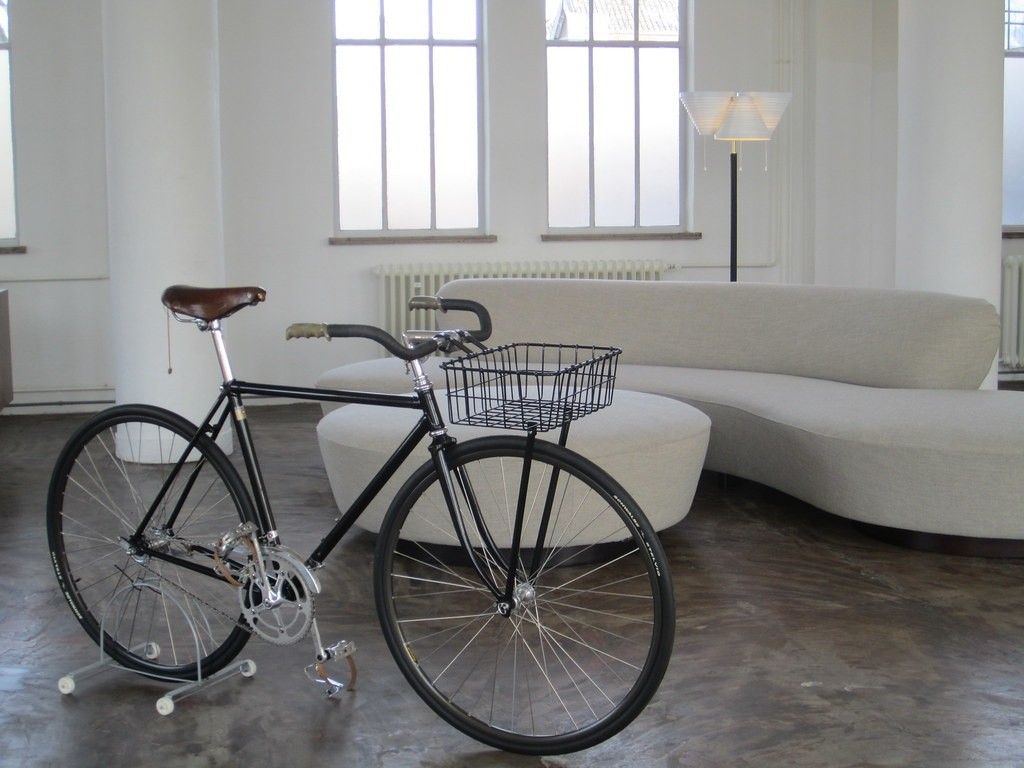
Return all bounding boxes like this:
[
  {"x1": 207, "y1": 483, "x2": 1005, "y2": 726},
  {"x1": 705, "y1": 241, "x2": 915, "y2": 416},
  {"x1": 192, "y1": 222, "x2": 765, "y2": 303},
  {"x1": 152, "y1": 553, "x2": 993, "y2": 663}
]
[{"x1": 434, "y1": 329, "x2": 622, "y2": 432}]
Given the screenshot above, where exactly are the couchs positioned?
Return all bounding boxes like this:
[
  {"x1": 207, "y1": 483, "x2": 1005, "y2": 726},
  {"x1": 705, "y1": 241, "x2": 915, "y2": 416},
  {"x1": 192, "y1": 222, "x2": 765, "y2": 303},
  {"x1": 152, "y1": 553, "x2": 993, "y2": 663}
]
[{"x1": 315, "y1": 276, "x2": 1024, "y2": 544}]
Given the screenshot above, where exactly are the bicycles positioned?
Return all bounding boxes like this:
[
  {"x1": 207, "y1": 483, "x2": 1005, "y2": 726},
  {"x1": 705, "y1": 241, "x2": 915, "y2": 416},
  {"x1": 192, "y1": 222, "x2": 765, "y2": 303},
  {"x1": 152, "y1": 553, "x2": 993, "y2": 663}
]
[{"x1": 44, "y1": 284, "x2": 677, "y2": 756}]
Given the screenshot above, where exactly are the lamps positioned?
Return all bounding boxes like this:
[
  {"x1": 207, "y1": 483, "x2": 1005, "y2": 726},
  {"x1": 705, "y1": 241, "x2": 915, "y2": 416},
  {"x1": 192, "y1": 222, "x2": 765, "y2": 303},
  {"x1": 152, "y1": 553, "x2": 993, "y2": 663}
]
[{"x1": 675, "y1": 89, "x2": 793, "y2": 283}]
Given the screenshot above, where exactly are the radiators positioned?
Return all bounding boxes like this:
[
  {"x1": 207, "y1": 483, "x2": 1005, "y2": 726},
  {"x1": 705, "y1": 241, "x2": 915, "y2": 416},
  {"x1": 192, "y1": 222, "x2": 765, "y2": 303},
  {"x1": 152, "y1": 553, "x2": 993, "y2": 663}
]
[{"x1": 373, "y1": 258, "x2": 687, "y2": 358}]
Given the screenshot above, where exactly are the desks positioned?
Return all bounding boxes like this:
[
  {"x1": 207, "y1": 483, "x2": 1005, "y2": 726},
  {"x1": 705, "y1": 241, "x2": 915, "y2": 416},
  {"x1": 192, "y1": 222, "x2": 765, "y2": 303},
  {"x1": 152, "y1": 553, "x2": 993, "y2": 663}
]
[{"x1": 318, "y1": 385, "x2": 712, "y2": 549}]
